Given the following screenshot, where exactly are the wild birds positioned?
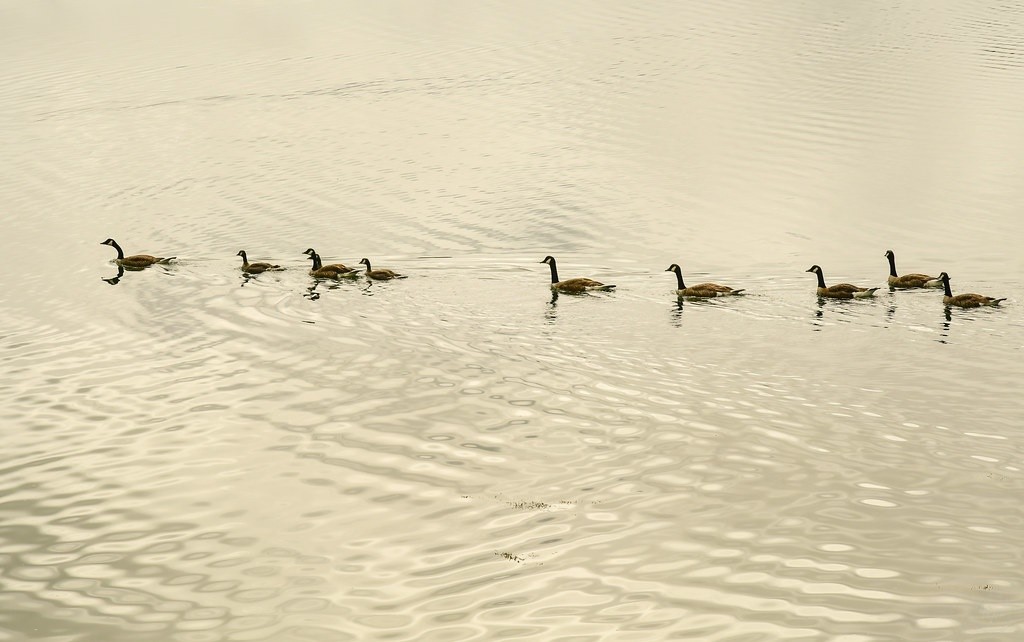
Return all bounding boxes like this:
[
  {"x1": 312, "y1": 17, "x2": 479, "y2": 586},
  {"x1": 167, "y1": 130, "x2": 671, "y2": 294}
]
[
  {"x1": 358, "y1": 257, "x2": 408, "y2": 281},
  {"x1": 663, "y1": 263, "x2": 746, "y2": 296},
  {"x1": 101, "y1": 264, "x2": 153, "y2": 286},
  {"x1": 99, "y1": 238, "x2": 178, "y2": 268},
  {"x1": 935, "y1": 272, "x2": 1009, "y2": 308},
  {"x1": 805, "y1": 264, "x2": 881, "y2": 298},
  {"x1": 539, "y1": 256, "x2": 617, "y2": 293},
  {"x1": 884, "y1": 249, "x2": 952, "y2": 290},
  {"x1": 302, "y1": 248, "x2": 363, "y2": 283},
  {"x1": 236, "y1": 250, "x2": 281, "y2": 273}
]
